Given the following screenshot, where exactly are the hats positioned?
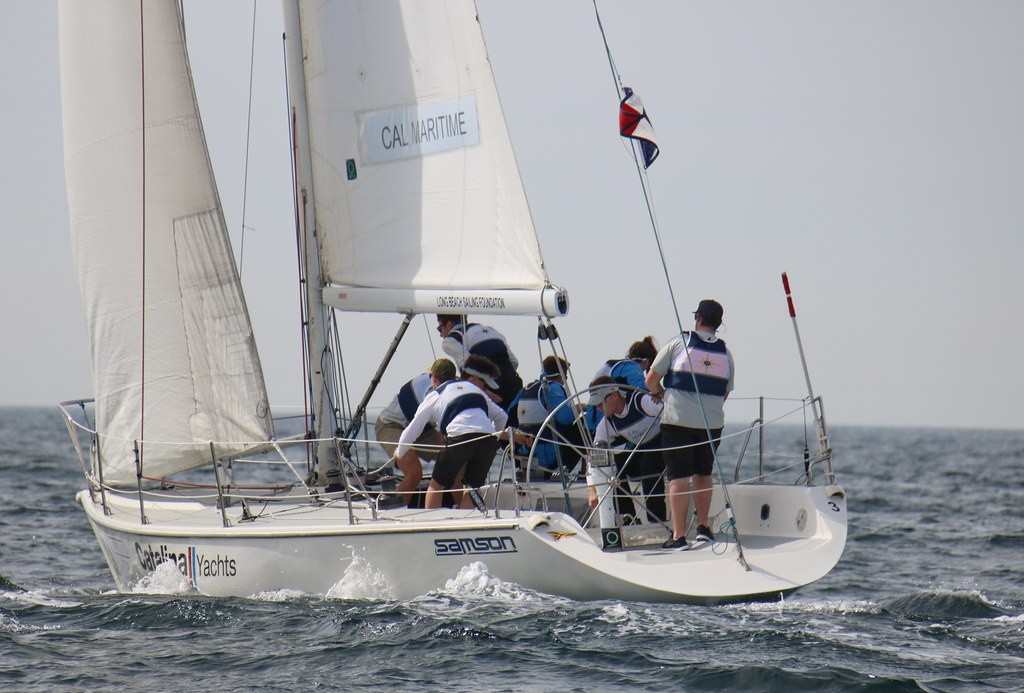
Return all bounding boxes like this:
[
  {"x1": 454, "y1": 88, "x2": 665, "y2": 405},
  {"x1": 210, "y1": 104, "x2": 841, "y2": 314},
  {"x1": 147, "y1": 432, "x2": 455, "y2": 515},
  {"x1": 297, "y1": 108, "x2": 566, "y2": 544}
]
[
  {"x1": 462, "y1": 367, "x2": 499, "y2": 390},
  {"x1": 692, "y1": 300, "x2": 723, "y2": 320},
  {"x1": 587, "y1": 386, "x2": 619, "y2": 406},
  {"x1": 431, "y1": 358, "x2": 456, "y2": 379}
]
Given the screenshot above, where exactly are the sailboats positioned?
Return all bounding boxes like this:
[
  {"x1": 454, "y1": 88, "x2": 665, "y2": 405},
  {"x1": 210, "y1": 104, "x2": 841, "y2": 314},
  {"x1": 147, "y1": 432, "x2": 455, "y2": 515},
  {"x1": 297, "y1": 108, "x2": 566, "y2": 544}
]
[{"x1": 54, "y1": 1, "x2": 849, "y2": 607}]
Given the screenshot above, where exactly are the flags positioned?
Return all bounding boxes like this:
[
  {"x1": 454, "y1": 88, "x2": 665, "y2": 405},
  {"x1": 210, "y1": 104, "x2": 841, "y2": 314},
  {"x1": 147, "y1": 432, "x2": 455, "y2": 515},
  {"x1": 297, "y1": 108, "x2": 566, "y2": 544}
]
[{"x1": 618, "y1": 87, "x2": 660, "y2": 170}]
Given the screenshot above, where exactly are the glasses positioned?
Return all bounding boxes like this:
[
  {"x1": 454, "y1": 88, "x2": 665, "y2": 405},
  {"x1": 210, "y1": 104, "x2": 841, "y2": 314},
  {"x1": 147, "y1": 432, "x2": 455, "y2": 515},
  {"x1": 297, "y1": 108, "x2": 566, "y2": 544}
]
[{"x1": 437, "y1": 320, "x2": 448, "y2": 333}]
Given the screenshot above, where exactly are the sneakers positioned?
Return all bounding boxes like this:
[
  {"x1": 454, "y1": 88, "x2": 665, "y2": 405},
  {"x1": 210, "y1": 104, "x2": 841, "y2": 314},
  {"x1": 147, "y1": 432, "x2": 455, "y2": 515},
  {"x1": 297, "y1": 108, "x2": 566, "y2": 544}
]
[
  {"x1": 696, "y1": 525, "x2": 715, "y2": 542},
  {"x1": 661, "y1": 534, "x2": 689, "y2": 551}
]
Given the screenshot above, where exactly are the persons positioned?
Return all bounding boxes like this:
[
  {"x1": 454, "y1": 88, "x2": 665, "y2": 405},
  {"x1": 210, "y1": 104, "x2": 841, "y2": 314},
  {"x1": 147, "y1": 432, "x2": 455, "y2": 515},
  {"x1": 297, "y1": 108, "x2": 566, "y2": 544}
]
[
  {"x1": 374, "y1": 314, "x2": 597, "y2": 509},
  {"x1": 645, "y1": 299, "x2": 734, "y2": 552},
  {"x1": 583, "y1": 335, "x2": 667, "y2": 526}
]
